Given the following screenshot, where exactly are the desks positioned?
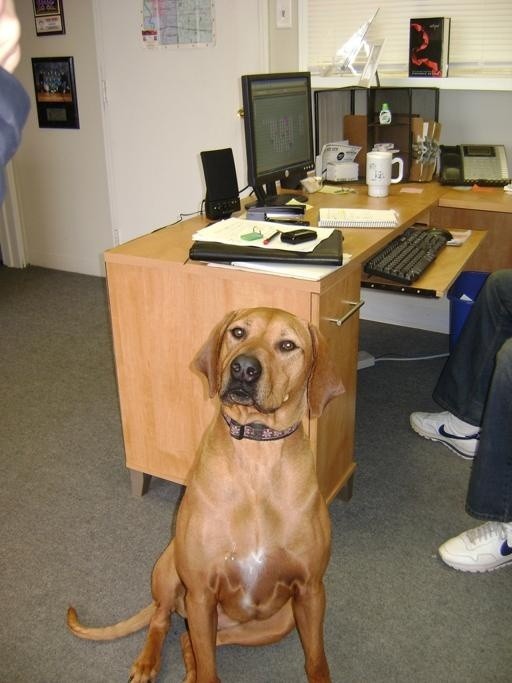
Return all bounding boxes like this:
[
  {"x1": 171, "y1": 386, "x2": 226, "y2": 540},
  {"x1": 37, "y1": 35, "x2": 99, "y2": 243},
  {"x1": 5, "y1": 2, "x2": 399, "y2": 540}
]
[{"x1": 103, "y1": 178, "x2": 512, "y2": 496}]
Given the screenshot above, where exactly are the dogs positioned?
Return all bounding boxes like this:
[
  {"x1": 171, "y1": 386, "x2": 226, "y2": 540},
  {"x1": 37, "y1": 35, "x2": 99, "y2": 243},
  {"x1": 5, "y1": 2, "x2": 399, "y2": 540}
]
[{"x1": 65, "y1": 306, "x2": 347, "y2": 683}]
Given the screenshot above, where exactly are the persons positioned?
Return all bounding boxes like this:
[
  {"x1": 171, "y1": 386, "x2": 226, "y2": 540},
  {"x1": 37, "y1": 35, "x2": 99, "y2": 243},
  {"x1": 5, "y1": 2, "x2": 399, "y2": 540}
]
[
  {"x1": 37, "y1": 70, "x2": 70, "y2": 94},
  {"x1": 1, "y1": 0, "x2": 33, "y2": 208},
  {"x1": 409, "y1": 265, "x2": 512, "y2": 573}
]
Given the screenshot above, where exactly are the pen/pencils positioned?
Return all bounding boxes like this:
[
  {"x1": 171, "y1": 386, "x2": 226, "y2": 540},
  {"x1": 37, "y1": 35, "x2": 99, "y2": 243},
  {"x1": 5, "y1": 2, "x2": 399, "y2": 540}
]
[{"x1": 264, "y1": 231, "x2": 280, "y2": 244}]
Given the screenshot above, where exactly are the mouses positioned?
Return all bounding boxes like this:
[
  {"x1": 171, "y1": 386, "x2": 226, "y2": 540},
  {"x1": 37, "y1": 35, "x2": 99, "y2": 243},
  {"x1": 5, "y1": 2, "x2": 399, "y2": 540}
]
[{"x1": 424, "y1": 227, "x2": 453, "y2": 241}]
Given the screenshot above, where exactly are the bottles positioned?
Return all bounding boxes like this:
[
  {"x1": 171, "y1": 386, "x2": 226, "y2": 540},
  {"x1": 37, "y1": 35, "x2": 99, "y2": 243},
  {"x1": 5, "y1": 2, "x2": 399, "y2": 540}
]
[{"x1": 378, "y1": 103, "x2": 391, "y2": 125}]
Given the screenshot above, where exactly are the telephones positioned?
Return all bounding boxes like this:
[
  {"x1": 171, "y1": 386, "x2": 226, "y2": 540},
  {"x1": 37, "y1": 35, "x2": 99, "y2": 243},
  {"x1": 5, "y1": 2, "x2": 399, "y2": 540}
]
[{"x1": 439, "y1": 144, "x2": 511, "y2": 186}]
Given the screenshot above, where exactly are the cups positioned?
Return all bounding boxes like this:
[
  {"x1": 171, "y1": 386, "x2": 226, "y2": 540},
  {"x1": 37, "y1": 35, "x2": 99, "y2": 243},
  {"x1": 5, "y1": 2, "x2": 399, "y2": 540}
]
[{"x1": 368, "y1": 152, "x2": 404, "y2": 198}]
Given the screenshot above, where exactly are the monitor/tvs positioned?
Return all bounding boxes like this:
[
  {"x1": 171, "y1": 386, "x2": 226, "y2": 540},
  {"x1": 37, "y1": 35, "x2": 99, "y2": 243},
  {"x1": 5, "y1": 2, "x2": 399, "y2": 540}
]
[{"x1": 241, "y1": 72, "x2": 315, "y2": 210}]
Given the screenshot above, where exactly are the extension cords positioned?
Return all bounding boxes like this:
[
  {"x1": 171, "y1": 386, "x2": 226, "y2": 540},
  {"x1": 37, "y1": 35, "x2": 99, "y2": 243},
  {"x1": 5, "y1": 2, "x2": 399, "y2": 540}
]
[{"x1": 357, "y1": 351, "x2": 376, "y2": 372}]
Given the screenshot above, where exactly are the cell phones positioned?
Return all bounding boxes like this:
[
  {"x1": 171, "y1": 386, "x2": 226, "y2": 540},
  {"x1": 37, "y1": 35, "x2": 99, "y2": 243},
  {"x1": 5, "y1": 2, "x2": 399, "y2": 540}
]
[{"x1": 281, "y1": 229, "x2": 317, "y2": 245}]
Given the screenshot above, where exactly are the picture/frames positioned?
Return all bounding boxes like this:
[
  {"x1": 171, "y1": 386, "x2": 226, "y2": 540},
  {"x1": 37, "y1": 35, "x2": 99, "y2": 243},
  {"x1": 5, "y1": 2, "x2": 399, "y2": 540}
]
[
  {"x1": 32, "y1": 0, "x2": 66, "y2": 36},
  {"x1": 32, "y1": 56, "x2": 80, "y2": 129}
]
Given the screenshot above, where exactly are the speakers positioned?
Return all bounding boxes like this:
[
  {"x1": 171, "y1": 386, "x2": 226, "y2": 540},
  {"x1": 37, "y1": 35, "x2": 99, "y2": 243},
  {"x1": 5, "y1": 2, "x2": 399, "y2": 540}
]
[
  {"x1": 199, "y1": 148, "x2": 241, "y2": 219},
  {"x1": 280, "y1": 170, "x2": 308, "y2": 191}
]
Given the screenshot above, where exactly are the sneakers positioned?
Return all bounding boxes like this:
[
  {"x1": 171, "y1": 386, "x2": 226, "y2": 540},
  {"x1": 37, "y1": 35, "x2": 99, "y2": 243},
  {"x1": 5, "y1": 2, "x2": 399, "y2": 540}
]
[
  {"x1": 408, "y1": 406, "x2": 482, "y2": 463},
  {"x1": 434, "y1": 512, "x2": 512, "y2": 579}
]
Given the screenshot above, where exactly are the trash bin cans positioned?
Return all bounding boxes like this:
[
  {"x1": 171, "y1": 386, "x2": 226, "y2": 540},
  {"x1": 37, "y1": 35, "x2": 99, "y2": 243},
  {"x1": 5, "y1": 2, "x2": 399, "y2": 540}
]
[{"x1": 447, "y1": 270, "x2": 490, "y2": 355}]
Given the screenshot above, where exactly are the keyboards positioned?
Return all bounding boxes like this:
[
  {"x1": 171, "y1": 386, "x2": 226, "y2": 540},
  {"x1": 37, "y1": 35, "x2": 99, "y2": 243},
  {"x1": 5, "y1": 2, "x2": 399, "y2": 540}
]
[{"x1": 365, "y1": 228, "x2": 446, "y2": 285}]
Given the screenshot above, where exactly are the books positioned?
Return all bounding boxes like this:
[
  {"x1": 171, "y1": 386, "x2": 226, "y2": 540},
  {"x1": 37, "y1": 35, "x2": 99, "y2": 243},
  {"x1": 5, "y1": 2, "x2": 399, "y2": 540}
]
[
  {"x1": 317, "y1": 206, "x2": 398, "y2": 227},
  {"x1": 187, "y1": 216, "x2": 352, "y2": 281},
  {"x1": 408, "y1": 16, "x2": 451, "y2": 77}
]
